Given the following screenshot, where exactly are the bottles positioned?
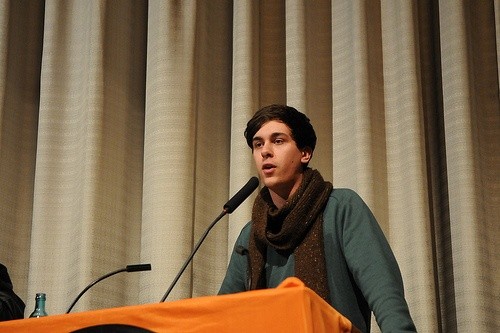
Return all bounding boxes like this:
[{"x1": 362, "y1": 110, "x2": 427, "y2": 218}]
[{"x1": 29, "y1": 293, "x2": 47, "y2": 318}]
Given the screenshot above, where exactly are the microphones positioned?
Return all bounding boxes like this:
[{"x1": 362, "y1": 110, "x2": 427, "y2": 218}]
[
  {"x1": 66, "y1": 263, "x2": 151, "y2": 315},
  {"x1": 160, "y1": 176, "x2": 260, "y2": 303}
]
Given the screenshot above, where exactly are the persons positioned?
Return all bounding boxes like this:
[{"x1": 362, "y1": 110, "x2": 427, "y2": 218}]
[
  {"x1": 217, "y1": 104, "x2": 416, "y2": 333},
  {"x1": 0, "y1": 263, "x2": 26, "y2": 323}
]
